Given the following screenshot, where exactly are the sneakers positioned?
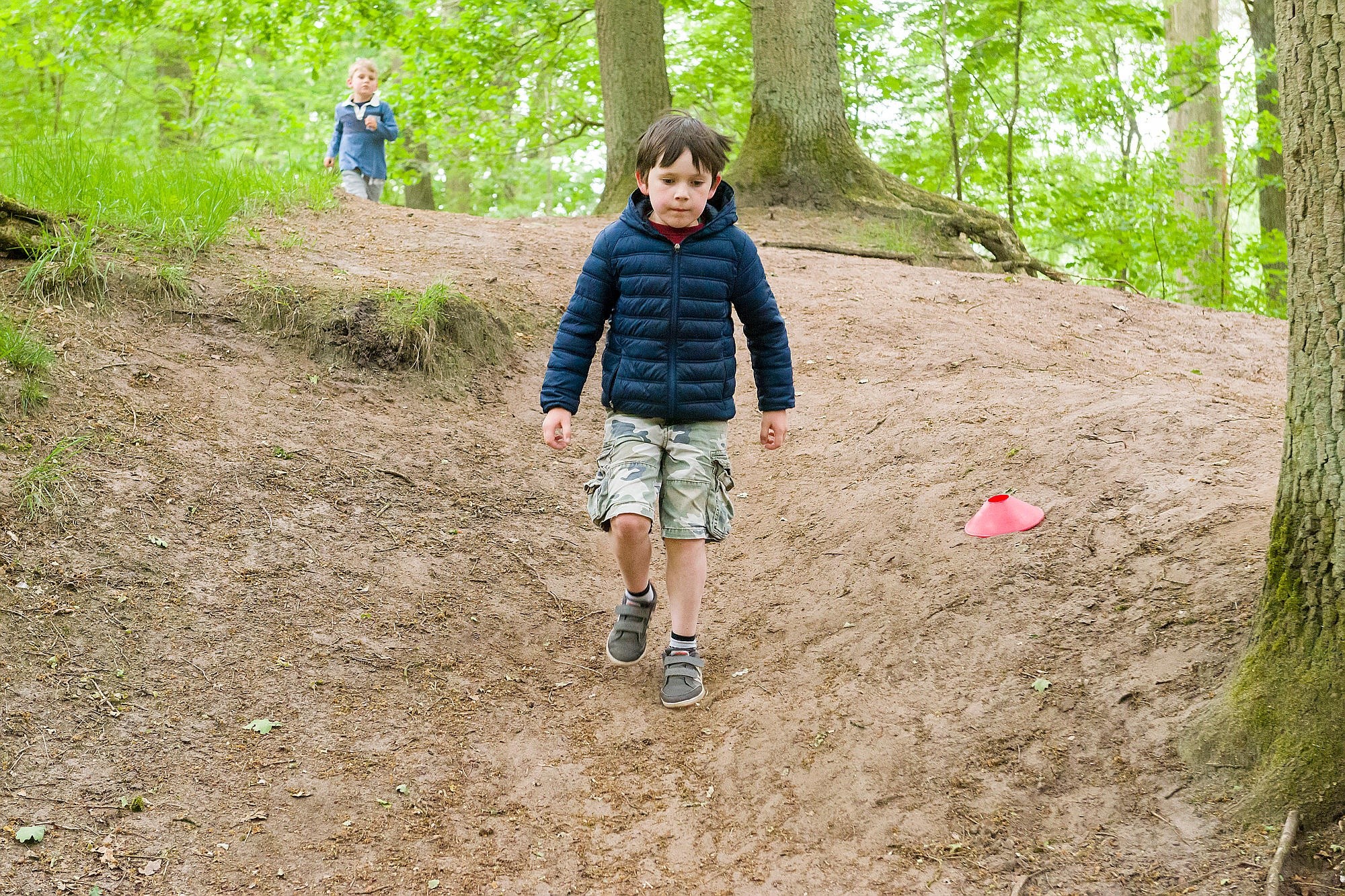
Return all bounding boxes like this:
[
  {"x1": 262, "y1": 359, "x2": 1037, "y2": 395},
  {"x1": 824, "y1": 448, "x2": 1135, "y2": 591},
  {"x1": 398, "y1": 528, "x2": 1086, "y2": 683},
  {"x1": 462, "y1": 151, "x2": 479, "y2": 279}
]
[
  {"x1": 606, "y1": 583, "x2": 657, "y2": 666},
  {"x1": 660, "y1": 648, "x2": 705, "y2": 708}
]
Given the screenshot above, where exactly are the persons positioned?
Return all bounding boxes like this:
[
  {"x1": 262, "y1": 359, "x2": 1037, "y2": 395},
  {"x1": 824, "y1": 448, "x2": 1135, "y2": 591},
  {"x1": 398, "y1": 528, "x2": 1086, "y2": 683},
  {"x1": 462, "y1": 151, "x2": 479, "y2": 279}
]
[
  {"x1": 324, "y1": 58, "x2": 398, "y2": 202},
  {"x1": 540, "y1": 111, "x2": 795, "y2": 707}
]
